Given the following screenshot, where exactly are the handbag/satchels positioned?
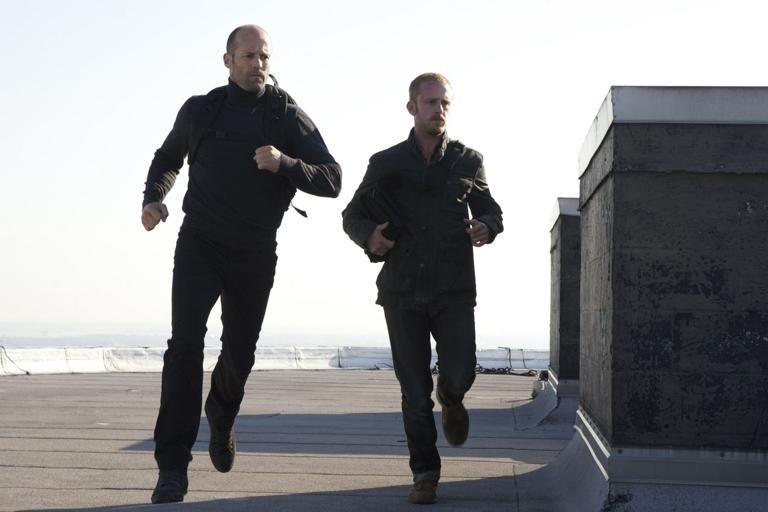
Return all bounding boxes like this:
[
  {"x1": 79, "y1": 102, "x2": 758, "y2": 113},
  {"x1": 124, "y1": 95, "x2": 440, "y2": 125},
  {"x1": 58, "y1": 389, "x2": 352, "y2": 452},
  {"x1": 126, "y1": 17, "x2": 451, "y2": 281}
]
[{"x1": 357, "y1": 174, "x2": 404, "y2": 263}]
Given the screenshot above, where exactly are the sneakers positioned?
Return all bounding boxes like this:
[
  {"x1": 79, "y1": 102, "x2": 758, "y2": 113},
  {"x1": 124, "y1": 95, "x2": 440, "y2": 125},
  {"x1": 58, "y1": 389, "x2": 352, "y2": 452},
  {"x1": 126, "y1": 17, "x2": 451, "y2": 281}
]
[
  {"x1": 436, "y1": 385, "x2": 469, "y2": 446},
  {"x1": 150, "y1": 469, "x2": 189, "y2": 504},
  {"x1": 204, "y1": 402, "x2": 236, "y2": 473},
  {"x1": 408, "y1": 471, "x2": 441, "y2": 503}
]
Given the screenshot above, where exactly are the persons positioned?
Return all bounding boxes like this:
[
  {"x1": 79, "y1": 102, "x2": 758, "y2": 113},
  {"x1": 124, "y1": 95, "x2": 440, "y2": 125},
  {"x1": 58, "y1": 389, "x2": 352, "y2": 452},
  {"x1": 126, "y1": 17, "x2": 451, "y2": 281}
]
[
  {"x1": 140, "y1": 24, "x2": 344, "y2": 505},
  {"x1": 341, "y1": 71, "x2": 504, "y2": 506}
]
